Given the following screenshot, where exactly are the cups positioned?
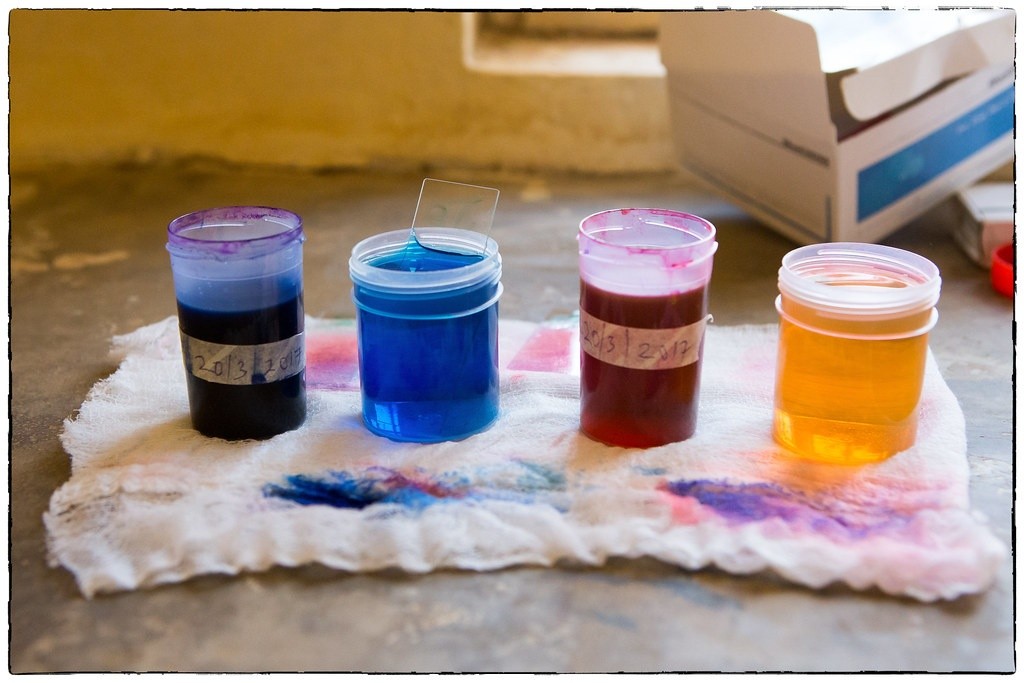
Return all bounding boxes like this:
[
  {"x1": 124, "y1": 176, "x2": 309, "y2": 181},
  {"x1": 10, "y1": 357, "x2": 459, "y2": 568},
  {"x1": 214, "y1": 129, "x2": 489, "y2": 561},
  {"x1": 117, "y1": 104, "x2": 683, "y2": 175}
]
[
  {"x1": 578, "y1": 206, "x2": 719, "y2": 448},
  {"x1": 773, "y1": 242, "x2": 943, "y2": 461},
  {"x1": 348, "y1": 228, "x2": 503, "y2": 444},
  {"x1": 166, "y1": 206, "x2": 306, "y2": 440}
]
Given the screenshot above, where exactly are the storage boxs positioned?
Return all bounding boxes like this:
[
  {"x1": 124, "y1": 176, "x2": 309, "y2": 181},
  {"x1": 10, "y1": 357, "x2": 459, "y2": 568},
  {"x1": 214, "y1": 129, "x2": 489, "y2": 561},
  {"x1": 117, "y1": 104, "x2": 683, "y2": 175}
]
[
  {"x1": 659, "y1": 11, "x2": 1015, "y2": 246},
  {"x1": 949, "y1": 181, "x2": 1014, "y2": 267}
]
